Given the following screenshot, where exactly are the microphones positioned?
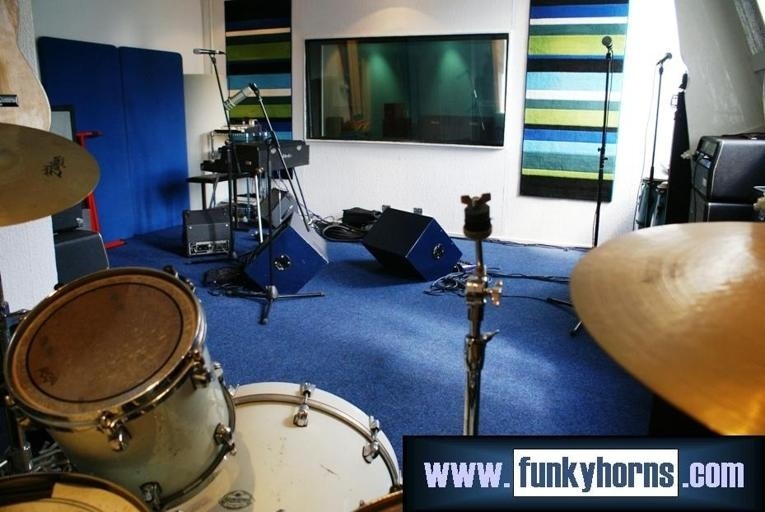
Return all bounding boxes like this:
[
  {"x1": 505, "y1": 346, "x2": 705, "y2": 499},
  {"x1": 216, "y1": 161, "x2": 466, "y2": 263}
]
[
  {"x1": 656, "y1": 52, "x2": 672, "y2": 66},
  {"x1": 193, "y1": 48, "x2": 224, "y2": 55},
  {"x1": 223, "y1": 86, "x2": 253, "y2": 111},
  {"x1": 602, "y1": 36, "x2": 613, "y2": 50}
]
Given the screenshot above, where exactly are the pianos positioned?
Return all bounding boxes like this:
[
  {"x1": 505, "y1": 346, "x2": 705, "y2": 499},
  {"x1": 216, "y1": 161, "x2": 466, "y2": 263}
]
[{"x1": 201, "y1": 141, "x2": 310, "y2": 172}]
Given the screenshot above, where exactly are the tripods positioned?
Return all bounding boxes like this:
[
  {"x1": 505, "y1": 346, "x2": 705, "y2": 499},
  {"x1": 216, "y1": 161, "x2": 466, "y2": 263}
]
[
  {"x1": 546, "y1": 54, "x2": 612, "y2": 336},
  {"x1": 185, "y1": 158, "x2": 254, "y2": 267}
]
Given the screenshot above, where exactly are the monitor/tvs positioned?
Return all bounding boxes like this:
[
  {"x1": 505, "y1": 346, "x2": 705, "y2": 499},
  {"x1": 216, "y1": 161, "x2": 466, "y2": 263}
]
[{"x1": 303, "y1": 33, "x2": 509, "y2": 149}]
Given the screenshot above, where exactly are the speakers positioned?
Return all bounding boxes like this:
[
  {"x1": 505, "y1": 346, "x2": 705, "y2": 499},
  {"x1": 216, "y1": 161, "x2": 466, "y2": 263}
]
[
  {"x1": 183, "y1": 207, "x2": 231, "y2": 257},
  {"x1": 361, "y1": 207, "x2": 463, "y2": 281},
  {"x1": 256, "y1": 188, "x2": 296, "y2": 228},
  {"x1": 53, "y1": 228, "x2": 111, "y2": 285},
  {"x1": 52, "y1": 194, "x2": 84, "y2": 233},
  {"x1": 342, "y1": 207, "x2": 372, "y2": 228},
  {"x1": 244, "y1": 211, "x2": 330, "y2": 296}
]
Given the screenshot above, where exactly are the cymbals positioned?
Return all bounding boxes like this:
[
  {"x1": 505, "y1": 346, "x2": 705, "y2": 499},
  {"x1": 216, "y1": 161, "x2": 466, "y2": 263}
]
[
  {"x1": 0, "y1": 121, "x2": 102, "y2": 227},
  {"x1": 572, "y1": 222, "x2": 765, "y2": 439}
]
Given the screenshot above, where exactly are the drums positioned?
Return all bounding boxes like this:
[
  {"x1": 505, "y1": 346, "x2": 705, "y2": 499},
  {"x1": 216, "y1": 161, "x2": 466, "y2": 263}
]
[
  {"x1": 155, "y1": 382, "x2": 403, "y2": 506},
  {"x1": 2, "y1": 472, "x2": 148, "y2": 506},
  {"x1": 2, "y1": 265, "x2": 237, "y2": 505},
  {"x1": 639, "y1": 177, "x2": 673, "y2": 229}
]
